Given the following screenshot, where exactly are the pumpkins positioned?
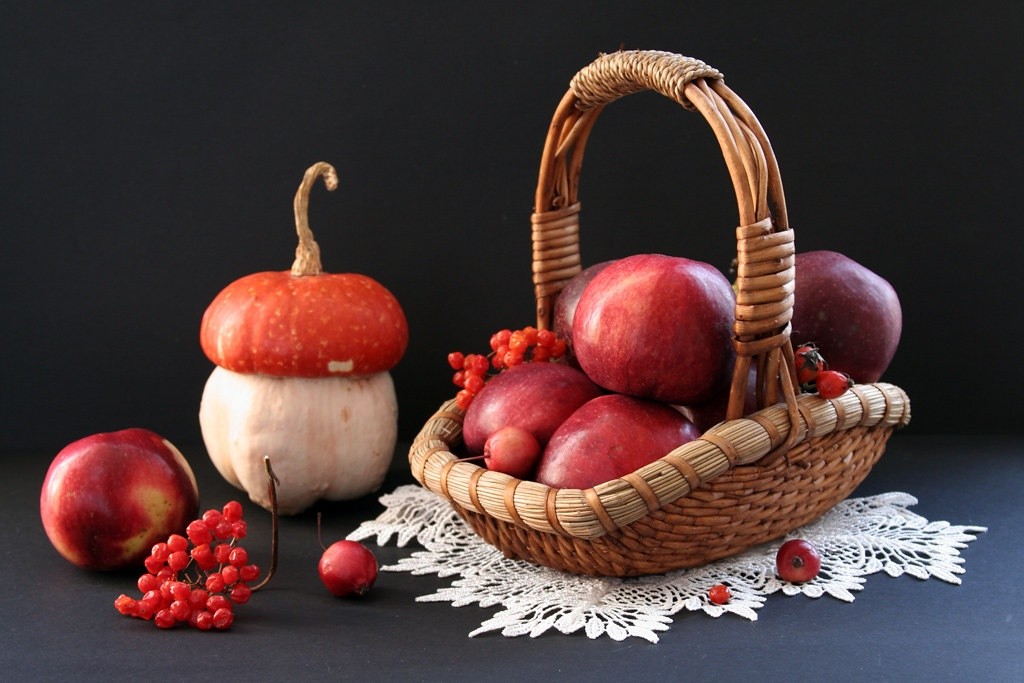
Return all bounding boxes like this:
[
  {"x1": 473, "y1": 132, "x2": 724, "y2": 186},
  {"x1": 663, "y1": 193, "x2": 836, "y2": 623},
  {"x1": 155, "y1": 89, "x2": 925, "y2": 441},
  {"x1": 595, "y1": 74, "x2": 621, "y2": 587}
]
[{"x1": 195, "y1": 161, "x2": 408, "y2": 386}]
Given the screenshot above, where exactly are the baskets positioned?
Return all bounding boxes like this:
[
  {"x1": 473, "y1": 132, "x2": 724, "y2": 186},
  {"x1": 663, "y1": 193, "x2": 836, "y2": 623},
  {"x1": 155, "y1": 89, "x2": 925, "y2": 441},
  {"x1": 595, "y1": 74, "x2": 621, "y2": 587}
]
[{"x1": 407, "y1": 50, "x2": 915, "y2": 582}]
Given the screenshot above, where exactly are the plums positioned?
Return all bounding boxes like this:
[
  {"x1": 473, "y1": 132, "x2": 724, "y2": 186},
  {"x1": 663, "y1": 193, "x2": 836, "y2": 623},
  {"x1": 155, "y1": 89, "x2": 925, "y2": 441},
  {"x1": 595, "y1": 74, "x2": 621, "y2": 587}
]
[{"x1": 40, "y1": 428, "x2": 201, "y2": 576}]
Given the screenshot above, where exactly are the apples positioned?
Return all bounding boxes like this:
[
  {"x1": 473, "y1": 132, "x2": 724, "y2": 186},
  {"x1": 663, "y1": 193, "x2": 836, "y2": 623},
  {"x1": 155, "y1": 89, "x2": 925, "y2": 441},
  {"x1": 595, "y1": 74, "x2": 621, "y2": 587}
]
[{"x1": 462, "y1": 247, "x2": 907, "y2": 491}]
